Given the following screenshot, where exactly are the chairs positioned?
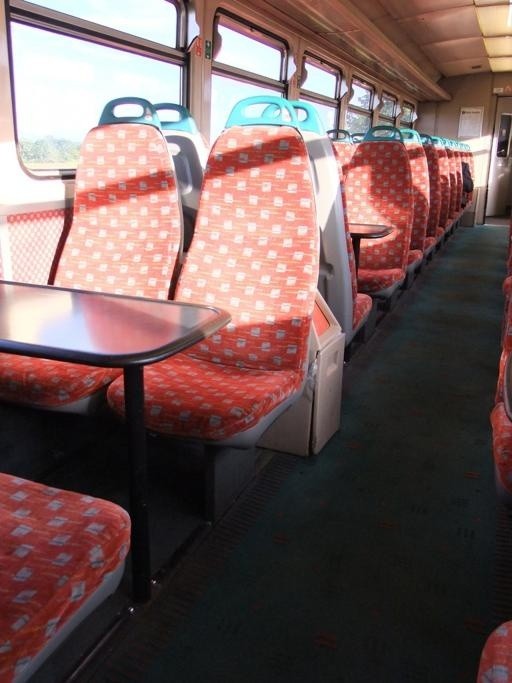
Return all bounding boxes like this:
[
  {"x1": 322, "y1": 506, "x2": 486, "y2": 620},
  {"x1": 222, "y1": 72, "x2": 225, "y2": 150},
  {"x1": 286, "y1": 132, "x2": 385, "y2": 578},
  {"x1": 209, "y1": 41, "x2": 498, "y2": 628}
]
[
  {"x1": 477, "y1": 622, "x2": 512, "y2": 683},
  {"x1": 106, "y1": 94, "x2": 322, "y2": 448},
  {"x1": 0, "y1": 472, "x2": 133, "y2": 682},
  {"x1": 1, "y1": 97, "x2": 184, "y2": 415},
  {"x1": 490, "y1": 300, "x2": 512, "y2": 494}
]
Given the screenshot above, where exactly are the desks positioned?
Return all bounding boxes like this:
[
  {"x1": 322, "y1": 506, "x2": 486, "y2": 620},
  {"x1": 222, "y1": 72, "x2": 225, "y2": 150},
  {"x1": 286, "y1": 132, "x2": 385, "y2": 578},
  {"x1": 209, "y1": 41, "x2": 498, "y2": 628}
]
[{"x1": 0, "y1": 279, "x2": 232, "y2": 600}]
